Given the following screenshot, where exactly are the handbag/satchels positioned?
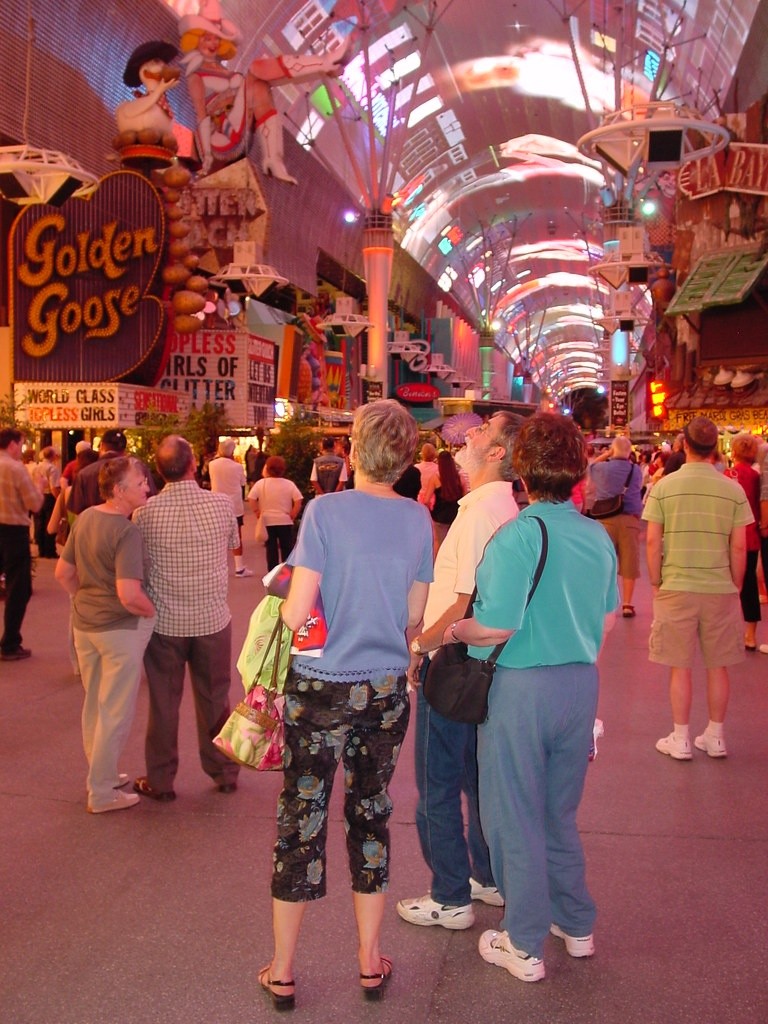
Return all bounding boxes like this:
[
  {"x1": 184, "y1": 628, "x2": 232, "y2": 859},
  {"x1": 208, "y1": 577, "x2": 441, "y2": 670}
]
[
  {"x1": 590, "y1": 494, "x2": 622, "y2": 520},
  {"x1": 423, "y1": 638, "x2": 494, "y2": 724},
  {"x1": 212, "y1": 618, "x2": 285, "y2": 772},
  {"x1": 55, "y1": 486, "x2": 72, "y2": 547},
  {"x1": 256, "y1": 516, "x2": 269, "y2": 544}
]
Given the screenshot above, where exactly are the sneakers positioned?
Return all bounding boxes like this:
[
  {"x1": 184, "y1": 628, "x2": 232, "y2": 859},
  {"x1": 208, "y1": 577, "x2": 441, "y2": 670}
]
[
  {"x1": 469, "y1": 877, "x2": 506, "y2": 906},
  {"x1": 397, "y1": 892, "x2": 476, "y2": 929},
  {"x1": 696, "y1": 730, "x2": 727, "y2": 757},
  {"x1": 551, "y1": 921, "x2": 596, "y2": 958},
  {"x1": 114, "y1": 772, "x2": 129, "y2": 789},
  {"x1": 656, "y1": 732, "x2": 693, "y2": 760},
  {"x1": 86, "y1": 788, "x2": 141, "y2": 813},
  {"x1": 478, "y1": 928, "x2": 545, "y2": 981}
]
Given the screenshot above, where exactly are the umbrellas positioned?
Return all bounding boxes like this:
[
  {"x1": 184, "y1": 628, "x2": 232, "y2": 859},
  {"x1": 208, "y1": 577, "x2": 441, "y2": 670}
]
[
  {"x1": 442, "y1": 413, "x2": 482, "y2": 444},
  {"x1": 587, "y1": 438, "x2": 614, "y2": 444}
]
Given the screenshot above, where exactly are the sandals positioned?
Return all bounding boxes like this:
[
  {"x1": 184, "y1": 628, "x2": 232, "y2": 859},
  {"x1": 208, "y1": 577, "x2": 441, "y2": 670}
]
[{"x1": 622, "y1": 604, "x2": 636, "y2": 618}]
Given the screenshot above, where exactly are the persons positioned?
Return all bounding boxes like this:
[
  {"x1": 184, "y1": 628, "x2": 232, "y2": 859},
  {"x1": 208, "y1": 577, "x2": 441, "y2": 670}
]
[
  {"x1": 178, "y1": 0, "x2": 350, "y2": 186},
  {"x1": 570, "y1": 416, "x2": 768, "y2": 759},
  {"x1": 0, "y1": 427, "x2": 247, "y2": 815},
  {"x1": 248, "y1": 400, "x2": 620, "y2": 1012}
]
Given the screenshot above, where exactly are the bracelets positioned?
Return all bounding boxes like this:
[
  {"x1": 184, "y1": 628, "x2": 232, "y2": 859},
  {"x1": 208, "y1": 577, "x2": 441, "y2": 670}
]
[{"x1": 451, "y1": 618, "x2": 465, "y2": 642}]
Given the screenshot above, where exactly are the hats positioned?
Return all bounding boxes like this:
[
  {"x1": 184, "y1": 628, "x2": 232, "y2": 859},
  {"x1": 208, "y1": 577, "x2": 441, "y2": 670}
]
[{"x1": 97, "y1": 429, "x2": 126, "y2": 449}]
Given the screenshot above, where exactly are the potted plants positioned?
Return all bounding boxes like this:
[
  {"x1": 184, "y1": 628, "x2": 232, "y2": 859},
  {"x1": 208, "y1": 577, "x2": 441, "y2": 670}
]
[{"x1": 269, "y1": 412, "x2": 319, "y2": 489}]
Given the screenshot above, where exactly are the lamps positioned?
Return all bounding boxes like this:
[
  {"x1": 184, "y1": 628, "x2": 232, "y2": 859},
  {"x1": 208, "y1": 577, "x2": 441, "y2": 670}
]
[{"x1": 0, "y1": 0, "x2": 753, "y2": 388}]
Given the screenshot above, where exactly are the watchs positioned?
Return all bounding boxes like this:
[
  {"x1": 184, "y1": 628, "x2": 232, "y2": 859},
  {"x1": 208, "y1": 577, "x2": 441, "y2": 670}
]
[{"x1": 411, "y1": 635, "x2": 428, "y2": 656}]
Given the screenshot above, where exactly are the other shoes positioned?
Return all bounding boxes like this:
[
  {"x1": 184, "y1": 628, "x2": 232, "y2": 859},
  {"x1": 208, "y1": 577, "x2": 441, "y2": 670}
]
[
  {"x1": 134, "y1": 775, "x2": 177, "y2": 801},
  {"x1": 235, "y1": 568, "x2": 254, "y2": 578},
  {"x1": 220, "y1": 783, "x2": 238, "y2": 793},
  {"x1": 0, "y1": 645, "x2": 33, "y2": 659}
]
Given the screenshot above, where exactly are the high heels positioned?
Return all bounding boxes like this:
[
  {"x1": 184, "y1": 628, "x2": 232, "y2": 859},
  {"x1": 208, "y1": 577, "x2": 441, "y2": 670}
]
[
  {"x1": 258, "y1": 962, "x2": 295, "y2": 1011},
  {"x1": 359, "y1": 958, "x2": 394, "y2": 1000}
]
